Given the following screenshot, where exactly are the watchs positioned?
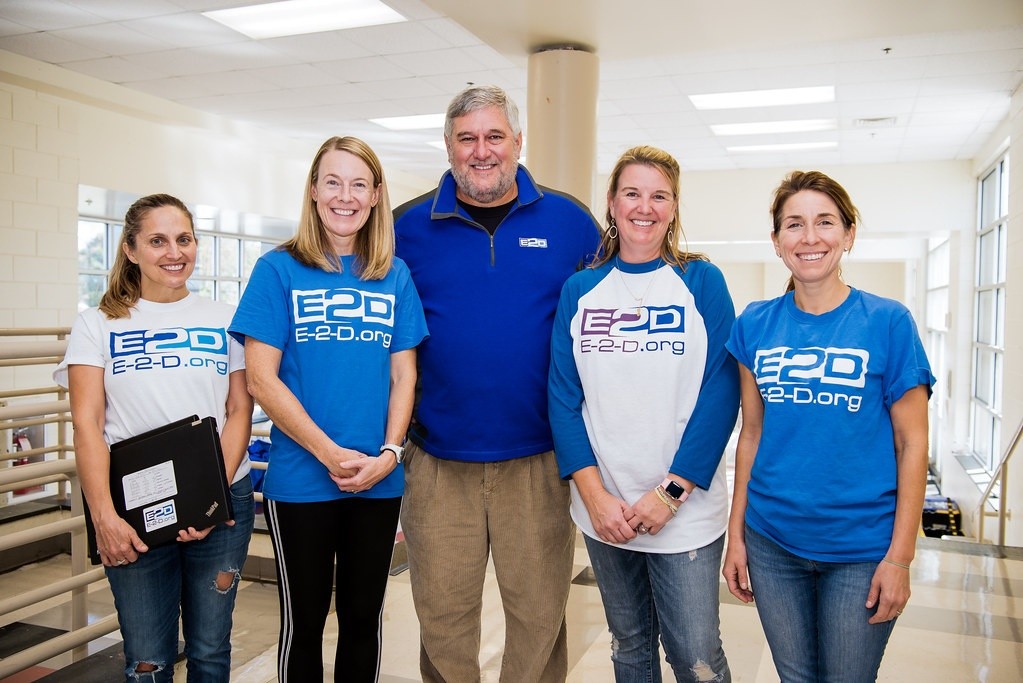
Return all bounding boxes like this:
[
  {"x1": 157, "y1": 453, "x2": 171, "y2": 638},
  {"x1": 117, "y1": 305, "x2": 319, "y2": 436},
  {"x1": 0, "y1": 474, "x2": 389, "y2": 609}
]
[
  {"x1": 380, "y1": 444, "x2": 407, "y2": 464},
  {"x1": 660, "y1": 478, "x2": 689, "y2": 503}
]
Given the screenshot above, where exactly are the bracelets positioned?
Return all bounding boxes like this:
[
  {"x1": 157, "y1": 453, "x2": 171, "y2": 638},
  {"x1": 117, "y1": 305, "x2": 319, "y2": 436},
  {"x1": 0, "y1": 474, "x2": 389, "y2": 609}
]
[
  {"x1": 883, "y1": 557, "x2": 909, "y2": 570},
  {"x1": 655, "y1": 486, "x2": 678, "y2": 517}
]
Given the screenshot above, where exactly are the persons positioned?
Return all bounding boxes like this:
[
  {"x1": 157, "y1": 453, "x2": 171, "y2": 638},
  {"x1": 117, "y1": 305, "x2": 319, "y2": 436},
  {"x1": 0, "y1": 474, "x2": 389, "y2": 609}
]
[
  {"x1": 722, "y1": 171, "x2": 936, "y2": 683},
  {"x1": 393, "y1": 86, "x2": 610, "y2": 683},
  {"x1": 546, "y1": 145, "x2": 743, "y2": 683},
  {"x1": 227, "y1": 136, "x2": 430, "y2": 682},
  {"x1": 53, "y1": 194, "x2": 254, "y2": 683}
]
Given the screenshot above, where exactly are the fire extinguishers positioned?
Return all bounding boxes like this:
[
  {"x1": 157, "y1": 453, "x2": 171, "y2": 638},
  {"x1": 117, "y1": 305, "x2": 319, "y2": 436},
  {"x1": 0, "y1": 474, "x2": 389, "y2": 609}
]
[{"x1": 12, "y1": 427, "x2": 44, "y2": 496}]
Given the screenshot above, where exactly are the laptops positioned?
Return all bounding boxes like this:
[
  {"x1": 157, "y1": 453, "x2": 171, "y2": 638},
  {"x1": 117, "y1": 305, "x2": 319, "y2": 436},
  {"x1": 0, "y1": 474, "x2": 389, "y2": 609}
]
[{"x1": 81, "y1": 414, "x2": 237, "y2": 565}]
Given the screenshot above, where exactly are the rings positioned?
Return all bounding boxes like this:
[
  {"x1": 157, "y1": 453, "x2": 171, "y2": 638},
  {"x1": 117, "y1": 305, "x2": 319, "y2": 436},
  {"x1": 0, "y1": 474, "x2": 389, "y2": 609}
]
[
  {"x1": 351, "y1": 488, "x2": 355, "y2": 493},
  {"x1": 896, "y1": 609, "x2": 902, "y2": 616},
  {"x1": 641, "y1": 525, "x2": 649, "y2": 534},
  {"x1": 117, "y1": 560, "x2": 126, "y2": 565}
]
